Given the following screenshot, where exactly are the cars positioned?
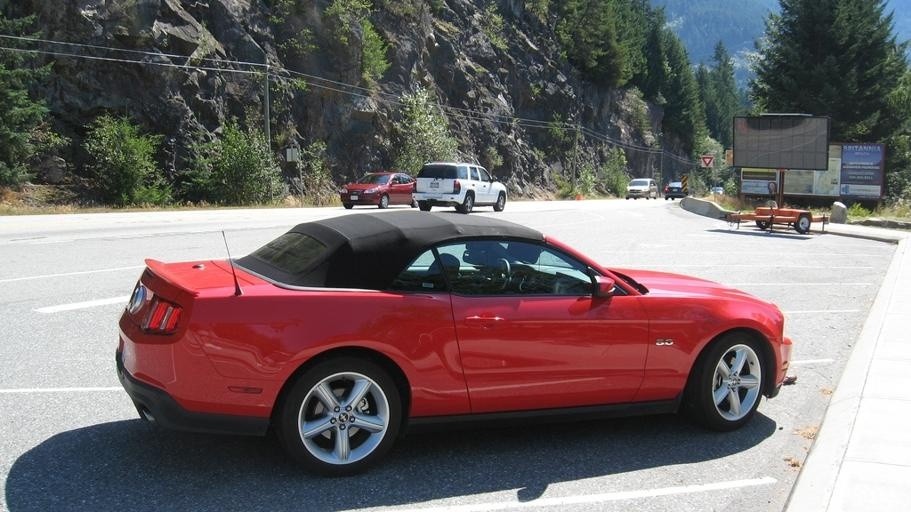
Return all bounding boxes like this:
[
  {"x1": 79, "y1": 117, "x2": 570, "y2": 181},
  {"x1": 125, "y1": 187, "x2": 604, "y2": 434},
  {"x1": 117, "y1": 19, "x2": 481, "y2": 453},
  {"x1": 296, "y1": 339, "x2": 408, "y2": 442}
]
[
  {"x1": 712, "y1": 186, "x2": 724, "y2": 192},
  {"x1": 664, "y1": 182, "x2": 687, "y2": 200},
  {"x1": 341, "y1": 173, "x2": 419, "y2": 208},
  {"x1": 625, "y1": 178, "x2": 659, "y2": 199}
]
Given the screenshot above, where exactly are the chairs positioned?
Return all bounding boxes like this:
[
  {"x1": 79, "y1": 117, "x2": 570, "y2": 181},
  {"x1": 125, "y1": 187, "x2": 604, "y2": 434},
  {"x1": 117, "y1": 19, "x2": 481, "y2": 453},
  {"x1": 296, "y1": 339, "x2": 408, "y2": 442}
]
[{"x1": 417, "y1": 254, "x2": 460, "y2": 292}]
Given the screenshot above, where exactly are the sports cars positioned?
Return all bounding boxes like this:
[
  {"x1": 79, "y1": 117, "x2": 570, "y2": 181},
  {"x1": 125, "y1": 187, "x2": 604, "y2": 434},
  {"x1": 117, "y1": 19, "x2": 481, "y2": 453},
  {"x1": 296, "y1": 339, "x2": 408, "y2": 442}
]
[{"x1": 116, "y1": 212, "x2": 792, "y2": 476}]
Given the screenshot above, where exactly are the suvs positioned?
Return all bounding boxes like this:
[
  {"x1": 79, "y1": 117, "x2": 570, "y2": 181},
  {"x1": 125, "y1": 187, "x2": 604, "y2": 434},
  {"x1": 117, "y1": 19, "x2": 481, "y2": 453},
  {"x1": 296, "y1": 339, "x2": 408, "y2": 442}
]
[{"x1": 411, "y1": 162, "x2": 508, "y2": 214}]
